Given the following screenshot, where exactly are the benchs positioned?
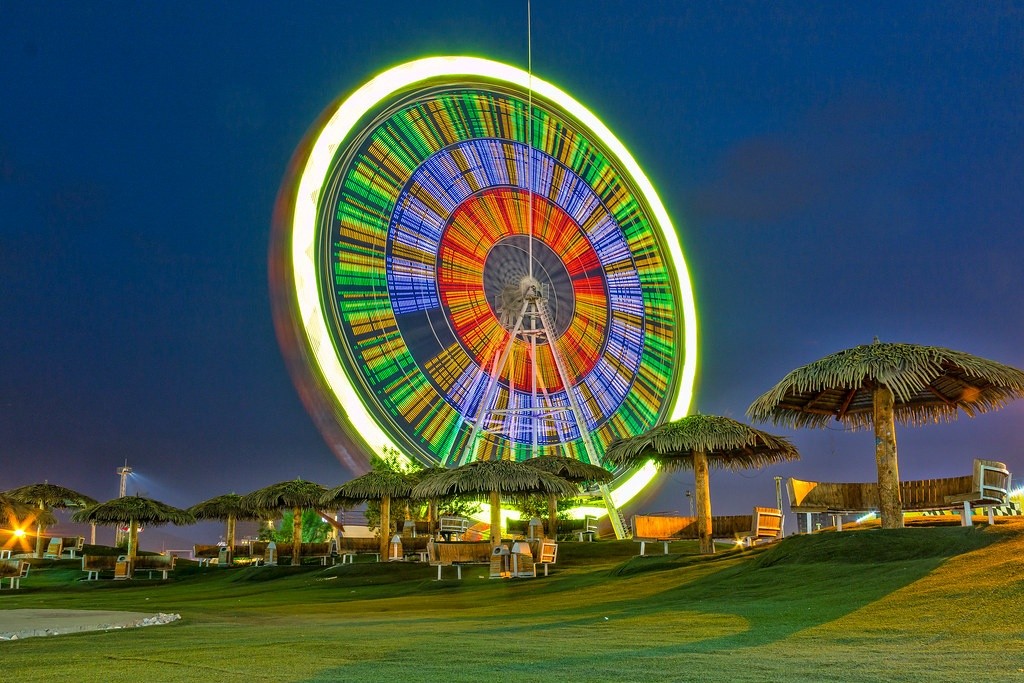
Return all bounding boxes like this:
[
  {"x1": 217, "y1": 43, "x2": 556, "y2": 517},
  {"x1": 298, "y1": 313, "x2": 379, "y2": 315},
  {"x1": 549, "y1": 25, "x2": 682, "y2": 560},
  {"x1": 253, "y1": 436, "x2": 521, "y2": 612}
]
[
  {"x1": 787, "y1": 457, "x2": 1012, "y2": 533},
  {"x1": 0, "y1": 535, "x2": 86, "y2": 559},
  {"x1": 193, "y1": 543, "x2": 249, "y2": 566},
  {"x1": 337, "y1": 535, "x2": 444, "y2": 562},
  {"x1": 630, "y1": 506, "x2": 784, "y2": 554},
  {"x1": 393, "y1": 520, "x2": 470, "y2": 540},
  {"x1": 426, "y1": 538, "x2": 559, "y2": 578},
  {"x1": 249, "y1": 540, "x2": 333, "y2": 566},
  {"x1": 506, "y1": 514, "x2": 598, "y2": 541},
  {"x1": 0, "y1": 558, "x2": 31, "y2": 588},
  {"x1": 81, "y1": 554, "x2": 179, "y2": 580}
]
